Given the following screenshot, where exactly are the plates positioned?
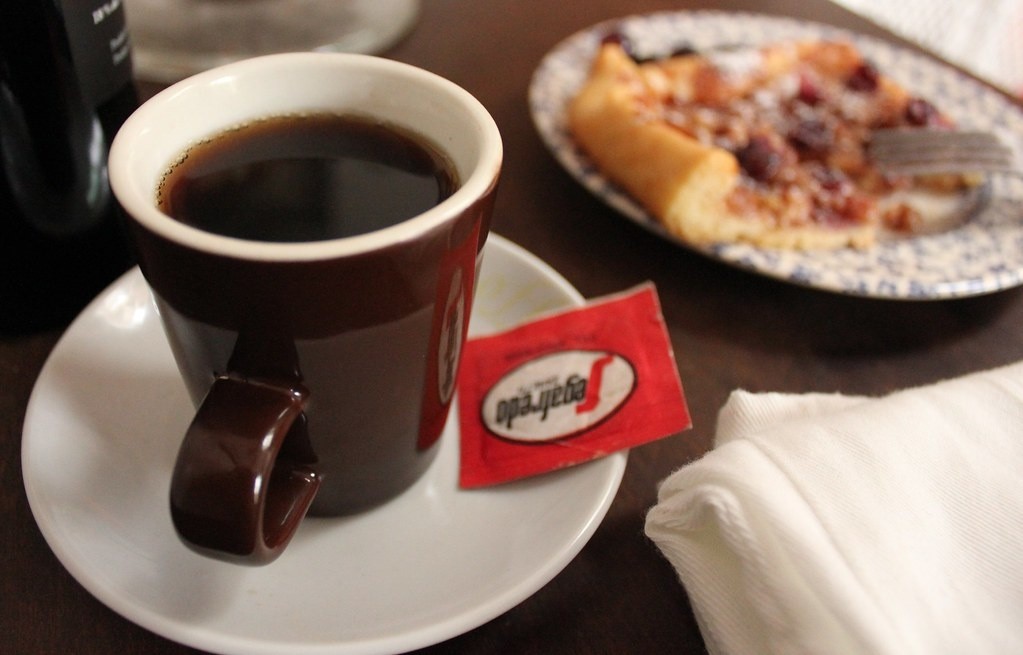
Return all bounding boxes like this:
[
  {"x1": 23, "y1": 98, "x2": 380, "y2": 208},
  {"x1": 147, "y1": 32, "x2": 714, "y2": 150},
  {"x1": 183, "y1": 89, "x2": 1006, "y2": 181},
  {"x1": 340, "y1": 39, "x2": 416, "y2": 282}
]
[
  {"x1": 124, "y1": 0, "x2": 422, "y2": 87},
  {"x1": 529, "y1": 7, "x2": 1022, "y2": 300},
  {"x1": 21, "y1": 229, "x2": 630, "y2": 655}
]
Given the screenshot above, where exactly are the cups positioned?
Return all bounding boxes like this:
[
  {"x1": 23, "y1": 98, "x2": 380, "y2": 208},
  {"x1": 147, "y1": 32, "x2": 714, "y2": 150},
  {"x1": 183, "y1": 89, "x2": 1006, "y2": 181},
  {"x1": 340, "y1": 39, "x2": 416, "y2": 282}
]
[{"x1": 107, "y1": 50, "x2": 506, "y2": 569}]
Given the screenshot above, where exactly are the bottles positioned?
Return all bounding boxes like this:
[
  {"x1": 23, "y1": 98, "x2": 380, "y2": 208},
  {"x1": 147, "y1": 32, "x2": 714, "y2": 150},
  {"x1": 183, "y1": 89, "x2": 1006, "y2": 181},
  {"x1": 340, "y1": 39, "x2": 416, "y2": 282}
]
[{"x1": 0, "y1": 2, "x2": 145, "y2": 340}]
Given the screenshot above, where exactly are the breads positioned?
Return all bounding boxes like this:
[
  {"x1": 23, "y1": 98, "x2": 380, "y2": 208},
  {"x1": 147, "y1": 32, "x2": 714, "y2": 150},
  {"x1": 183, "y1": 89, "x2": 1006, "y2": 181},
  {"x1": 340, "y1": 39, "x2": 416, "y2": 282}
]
[{"x1": 571, "y1": 42, "x2": 983, "y2": 247}]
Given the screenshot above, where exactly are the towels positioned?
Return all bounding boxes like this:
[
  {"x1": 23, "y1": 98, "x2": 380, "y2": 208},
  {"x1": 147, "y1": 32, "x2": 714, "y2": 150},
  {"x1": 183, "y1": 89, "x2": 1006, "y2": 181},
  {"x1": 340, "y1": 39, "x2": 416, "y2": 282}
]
[{"x1": 642, "y1": 359, "x2": 1020, "y2": 654}]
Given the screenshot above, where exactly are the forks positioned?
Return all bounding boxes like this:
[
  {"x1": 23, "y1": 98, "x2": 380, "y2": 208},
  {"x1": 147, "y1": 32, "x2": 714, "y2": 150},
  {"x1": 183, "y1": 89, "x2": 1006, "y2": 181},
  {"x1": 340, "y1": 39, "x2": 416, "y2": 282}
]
[{"x1": 871, "y1": 123, "x2": 1023, "y2": 185}]
